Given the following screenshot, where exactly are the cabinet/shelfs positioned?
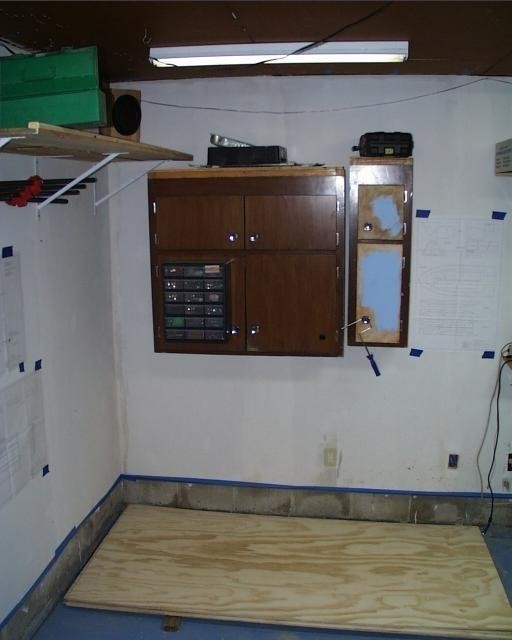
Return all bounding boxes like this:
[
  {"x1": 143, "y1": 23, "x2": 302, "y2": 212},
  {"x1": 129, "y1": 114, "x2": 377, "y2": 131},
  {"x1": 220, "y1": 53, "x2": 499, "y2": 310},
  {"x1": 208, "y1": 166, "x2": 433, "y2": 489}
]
[
  {"x1": 344, "y1": 159, "x2": 411, "y2": 349},
  {"x1": 146, "y1": 165, "x2": 346, "y2": 358}
]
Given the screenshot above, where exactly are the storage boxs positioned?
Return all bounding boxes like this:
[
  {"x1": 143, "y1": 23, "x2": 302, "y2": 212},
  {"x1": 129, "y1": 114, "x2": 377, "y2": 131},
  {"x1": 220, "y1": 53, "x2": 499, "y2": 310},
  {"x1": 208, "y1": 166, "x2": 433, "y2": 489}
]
[{"x1": 0, "y1": 44, "x2": 109, "y2": 132}]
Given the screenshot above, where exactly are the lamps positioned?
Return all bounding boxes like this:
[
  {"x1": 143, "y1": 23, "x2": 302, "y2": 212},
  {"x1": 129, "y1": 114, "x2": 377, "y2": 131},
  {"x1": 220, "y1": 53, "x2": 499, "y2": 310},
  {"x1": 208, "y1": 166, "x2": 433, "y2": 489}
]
[{"x1": 148, "y1": 40, "x2": 412, "y2": 69}]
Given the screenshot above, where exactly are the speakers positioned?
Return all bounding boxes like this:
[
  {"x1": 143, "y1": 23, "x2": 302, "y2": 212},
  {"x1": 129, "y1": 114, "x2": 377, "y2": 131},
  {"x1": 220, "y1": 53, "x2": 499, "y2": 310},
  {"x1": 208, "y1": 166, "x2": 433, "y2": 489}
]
[{"x1": 99, "y1": 87, "x2": 142, "y2": 142}]
